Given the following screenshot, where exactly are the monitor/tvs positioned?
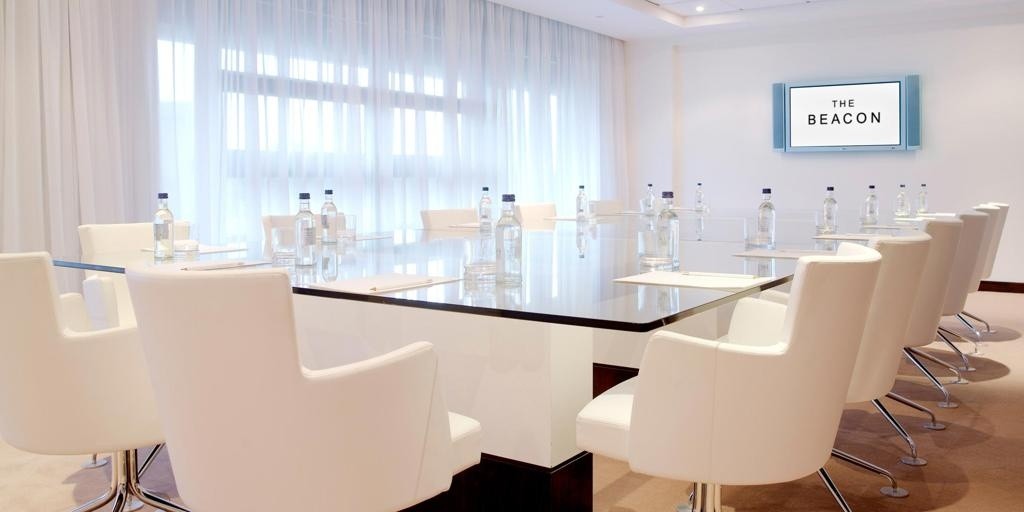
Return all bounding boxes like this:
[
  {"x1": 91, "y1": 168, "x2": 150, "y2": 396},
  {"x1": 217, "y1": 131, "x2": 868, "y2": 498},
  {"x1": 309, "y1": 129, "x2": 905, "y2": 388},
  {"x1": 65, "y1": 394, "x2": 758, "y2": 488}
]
[{"x1": 785, "y1": 76, "x2": 905, "y2": 152}]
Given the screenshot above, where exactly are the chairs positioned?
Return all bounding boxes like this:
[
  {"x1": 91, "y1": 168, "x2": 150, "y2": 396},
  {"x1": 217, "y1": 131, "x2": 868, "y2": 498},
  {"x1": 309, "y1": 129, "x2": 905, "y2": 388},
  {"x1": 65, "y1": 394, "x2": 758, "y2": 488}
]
[
  {"x1": 119, "y1": 262, "x2": 483, "y2": 510},
  {"x1": 1, "y1": 250, "x2": 186, "y2": 510},
  {"x1": 260, "y1": 200, "x2": 638, "y2": 254},
  {"x1": 761, "y1": 202, "x2": 1013, "y2": 498},
  {"x1": 574, "y1": 241, "x2": 883, "y2": 509},
  {"x1": 77, "y1": 221, "x2": 193, "y2": 326}
]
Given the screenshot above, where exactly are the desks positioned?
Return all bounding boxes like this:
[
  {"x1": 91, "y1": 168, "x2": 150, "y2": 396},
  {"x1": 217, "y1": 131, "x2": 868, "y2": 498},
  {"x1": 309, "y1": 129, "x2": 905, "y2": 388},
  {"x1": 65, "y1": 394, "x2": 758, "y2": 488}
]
[{"x1": 54, "y1": 210, "x2": 979, "y2": 509}]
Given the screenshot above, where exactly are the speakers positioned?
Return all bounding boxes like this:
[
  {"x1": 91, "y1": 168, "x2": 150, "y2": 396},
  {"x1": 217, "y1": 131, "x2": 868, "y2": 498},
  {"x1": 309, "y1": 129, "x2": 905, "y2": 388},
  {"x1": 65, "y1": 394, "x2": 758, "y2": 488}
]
[
  {"x1": 772, "y1": 82, "x2": 785, "y2": 152},
  {"x1": 906, "y1": 74, "x2": 921, "y2": 151}
]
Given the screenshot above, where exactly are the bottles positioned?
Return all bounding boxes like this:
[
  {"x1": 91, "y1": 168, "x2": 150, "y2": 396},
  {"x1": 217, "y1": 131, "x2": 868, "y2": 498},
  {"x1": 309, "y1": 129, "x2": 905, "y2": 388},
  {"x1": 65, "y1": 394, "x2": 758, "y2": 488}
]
[
  {"x1": 492, "y1": 193, "x2": 525, "y2": 288},
  {"x1": 695, "y1": 211, "x2": 704, "y2": 242},
  {"x1": 576, "y1": 222, "x2": 586, "y2": 260},
  {"x1": 295, "y1": 191, "x2": 315, "y2": 267},
  {"x1": 822, "y1": 185, "x2": 839, "y2": 233},
  {"x1": 320, "y1": 189, "x2": 338, "y2": 244},
  {"x1": 476, "y1": 180, "x2": 706, "y2": 232},
  {"x1": 492, "y1": 282, "x2": 523, "y2": 311},
  {"x1": 322, "y1": 242, "x2": 338, "y2": 286},
  {"x1": 657, "y1": 267, "x2": 681, "y2": 316},
  {"x1": 152, "y1": 192, "x2": 175, "y2": 261},
  {"x1": 654, "y1": 191, "x2": 681, "y2": 264},
  {"x1": 864, "y1": 183, "x2": 927, "y2": 223},
  {"x1": 758, "y1": 246, "x2": 774, "y2": 278},
  {"x1": 756, "y1": 186, "x2": 776, "y2": 249}
]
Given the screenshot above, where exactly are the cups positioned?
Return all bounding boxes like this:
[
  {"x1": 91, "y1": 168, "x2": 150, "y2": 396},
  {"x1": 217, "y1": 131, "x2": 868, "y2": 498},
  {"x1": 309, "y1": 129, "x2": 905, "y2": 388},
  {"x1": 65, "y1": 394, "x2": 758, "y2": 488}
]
[
  {"x1": 743, "y1": 246, "x2": 757, "y2": 275},
  {"x1": 587, "y1": 220, "x2": 599, "y2": 239},
  {"x1": 272, "y1": 256, "x2": 297, "y2": 283},
  {"x1": 636, "y1": 230, "x2": 673, "y2": 267},
  {"x1": 812, "y1": 233, "x2": 838, "y2": 253},
  {"x1": 637, "y1": 264, "x2": 657, "y2": 313},
  {"x1": 267, "y1": 225, "x2": 298, "y2": 259},
  {"x1": 742, "y1": 216, "x2": 769, "y2": 249},
  {"x1": 462, "y1": 236, "x2": 499, "y2": 282},
  {"x1": 814, "y1": 207, "x2": 835, "y2": 235},
  {"x1": 173, "y1": 222, "x2": 201, "y2": 258},
  {"x1": 704, "y1": 199, "x2": 712, "y2": 214},
  {"x1": 460, "y1": 283, "x2": 491, "y2": 308},
  {"x1": 336, "y1": 213, "x2": 357, "y2": 243}
]
